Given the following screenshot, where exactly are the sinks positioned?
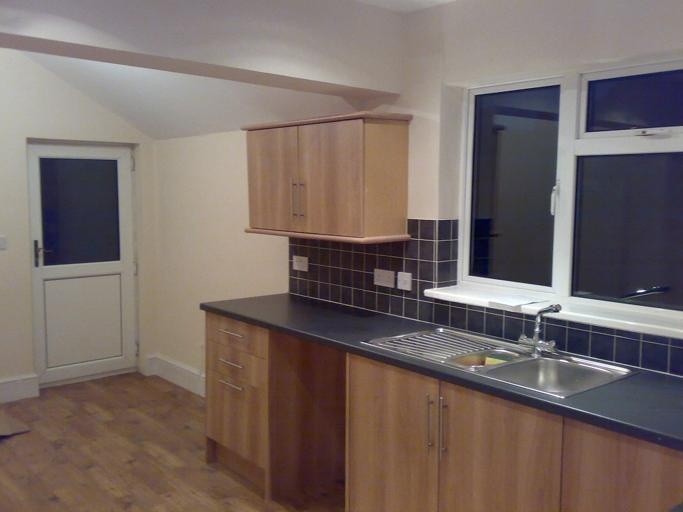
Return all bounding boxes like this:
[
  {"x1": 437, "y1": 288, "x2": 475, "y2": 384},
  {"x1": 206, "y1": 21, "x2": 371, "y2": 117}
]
[
  {"x1": 477, "y1": 357, "x2": 640, "y2": 399},
  {"x1": 445, "y1": 349, "x2": 537, "y2": 367}
]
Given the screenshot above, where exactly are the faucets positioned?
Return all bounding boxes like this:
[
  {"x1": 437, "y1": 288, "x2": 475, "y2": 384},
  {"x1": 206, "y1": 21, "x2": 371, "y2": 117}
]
[
  {"x1": 517, "y1": 303, "x2": 562, "y2": 358},
  {"x1": 620, "y1": 285, "x2": 669, "y2": 299}
]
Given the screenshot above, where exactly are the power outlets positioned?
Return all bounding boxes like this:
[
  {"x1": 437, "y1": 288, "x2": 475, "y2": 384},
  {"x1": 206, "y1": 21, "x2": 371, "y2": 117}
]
[
  {"x1": 292, "y1": 256, "x2": 309, "y2": 271},
  {"x1": 373, "y1": 269, "x2": 395, "y2": 289},
  {"x1": 397, "y1": 271, "x2": 412, "y2": 291}
]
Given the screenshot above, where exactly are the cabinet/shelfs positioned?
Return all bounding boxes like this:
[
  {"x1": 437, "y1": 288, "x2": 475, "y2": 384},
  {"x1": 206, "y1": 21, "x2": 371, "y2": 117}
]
[
  {"x1": 240, "y1": 111, "x2": 413, "y2": 244},
  {"x1": 206, "y1": 311, "x2": 683, "y2": 512}
]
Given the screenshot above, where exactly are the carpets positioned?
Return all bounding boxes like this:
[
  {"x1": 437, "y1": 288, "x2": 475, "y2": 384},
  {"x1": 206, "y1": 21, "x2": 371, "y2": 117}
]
[{"x1": 0, "y1": 406, "x2": 30, "y2": 437}]
